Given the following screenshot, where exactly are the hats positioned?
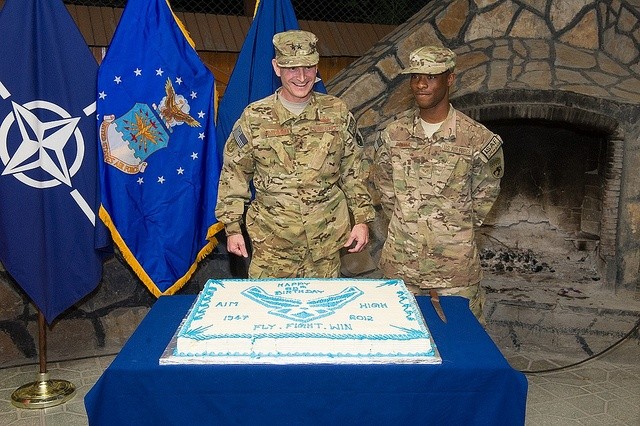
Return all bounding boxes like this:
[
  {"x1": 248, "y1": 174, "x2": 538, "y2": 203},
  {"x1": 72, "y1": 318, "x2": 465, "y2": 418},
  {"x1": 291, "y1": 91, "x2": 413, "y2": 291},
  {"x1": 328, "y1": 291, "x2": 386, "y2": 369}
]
[
  {"x1": 400, "y1": 45, "x2": 457, "y2": 75},
  {"x1": 272, "y1": 29, "x2": 320, "y2": 68}
]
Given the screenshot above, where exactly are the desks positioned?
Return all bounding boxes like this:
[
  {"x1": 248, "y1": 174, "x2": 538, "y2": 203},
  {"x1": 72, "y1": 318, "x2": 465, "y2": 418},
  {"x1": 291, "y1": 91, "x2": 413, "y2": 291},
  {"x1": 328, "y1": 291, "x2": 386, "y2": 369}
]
[{"x1": 82, "y1": 294, "x2": 528, "y2": 424}]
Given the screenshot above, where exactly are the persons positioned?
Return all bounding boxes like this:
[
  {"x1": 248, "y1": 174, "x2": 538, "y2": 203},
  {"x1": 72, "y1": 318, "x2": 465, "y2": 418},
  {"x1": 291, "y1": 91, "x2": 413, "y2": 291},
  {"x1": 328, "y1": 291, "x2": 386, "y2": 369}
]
[
  {"x1": 369, "y1": 44, "x2": 505, "y2": 330},
  {"x1": 213, "y1": 30, "x2": 375, "y2": 279}
]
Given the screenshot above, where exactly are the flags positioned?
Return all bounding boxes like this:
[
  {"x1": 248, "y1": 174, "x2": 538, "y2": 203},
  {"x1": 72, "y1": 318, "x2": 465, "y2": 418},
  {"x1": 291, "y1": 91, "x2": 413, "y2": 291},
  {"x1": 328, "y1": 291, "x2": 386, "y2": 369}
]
[
  {"x1": 215, "y1": 0, "x2": 327, "y2": 196},
  {"x1": 97, "y1": 0, "x2": 223, "y2": 299},
  {"x1": 0, "y1": 0, "x2": 110, "y2": 326}
]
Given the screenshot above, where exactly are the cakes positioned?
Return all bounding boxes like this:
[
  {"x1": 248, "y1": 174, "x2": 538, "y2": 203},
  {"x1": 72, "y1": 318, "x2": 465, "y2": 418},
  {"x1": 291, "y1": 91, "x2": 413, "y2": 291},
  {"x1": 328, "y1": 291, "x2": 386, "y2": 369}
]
[{"x1": 174, "y1": 277, "x2": 434, "y2": 359}]
